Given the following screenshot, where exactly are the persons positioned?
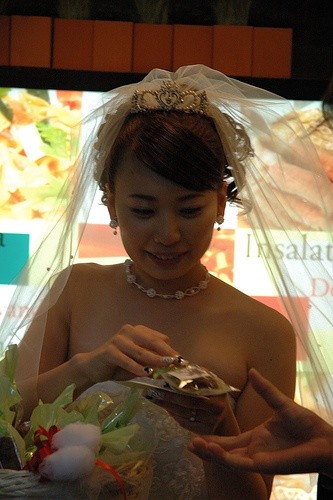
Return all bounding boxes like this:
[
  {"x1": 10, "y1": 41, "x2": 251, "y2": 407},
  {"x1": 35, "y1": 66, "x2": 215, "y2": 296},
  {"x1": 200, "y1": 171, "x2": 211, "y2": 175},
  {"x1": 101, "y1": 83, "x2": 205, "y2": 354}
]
[
  {"x1": 0, "y1": 64, "x2": 333, "y2": 500},
  {"x1": 185, "y1": 368, "x2": 333, "y2": 500}
]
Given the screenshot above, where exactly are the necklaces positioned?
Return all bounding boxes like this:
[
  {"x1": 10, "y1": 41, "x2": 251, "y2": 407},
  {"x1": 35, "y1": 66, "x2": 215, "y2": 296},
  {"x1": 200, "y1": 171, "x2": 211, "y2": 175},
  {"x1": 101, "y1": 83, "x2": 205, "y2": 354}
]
[{"x1": 125, "y1": 259, "x2": 212, "y2": 301}]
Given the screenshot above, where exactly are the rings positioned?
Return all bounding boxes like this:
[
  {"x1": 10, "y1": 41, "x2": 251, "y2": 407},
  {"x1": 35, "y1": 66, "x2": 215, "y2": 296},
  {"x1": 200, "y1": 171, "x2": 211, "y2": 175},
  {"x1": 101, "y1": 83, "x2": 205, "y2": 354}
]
[{"x1": 189, "y1": 409, "x2": 198, "y2": 422}]
